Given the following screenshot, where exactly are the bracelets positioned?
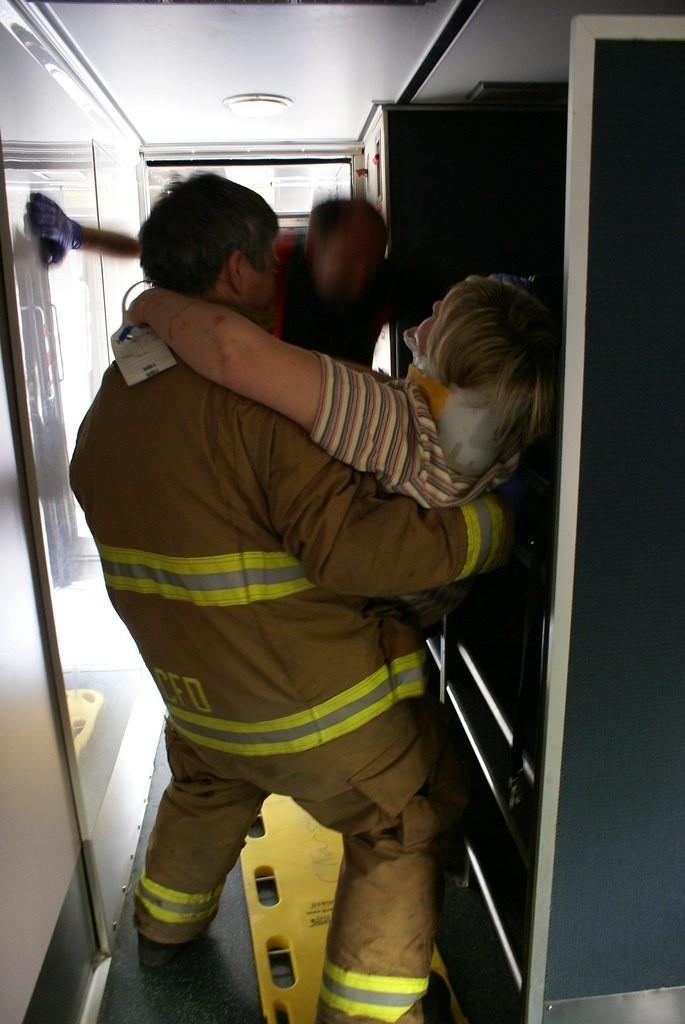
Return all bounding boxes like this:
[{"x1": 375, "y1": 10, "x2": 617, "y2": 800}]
[{"x1": 122, "y1": 278, "x2": 159, "y2": 330}]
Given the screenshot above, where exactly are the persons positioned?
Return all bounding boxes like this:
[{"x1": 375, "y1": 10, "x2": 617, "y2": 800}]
[
  {"x1": 26, "y1": 190, "x2": 558, "y2": 369},
  {"x1": 64, "y1": 169, "x2": 515, "y2": 1024},
  {"x1": 119, "y1": 276, "x2": 563, "y2": 513}
]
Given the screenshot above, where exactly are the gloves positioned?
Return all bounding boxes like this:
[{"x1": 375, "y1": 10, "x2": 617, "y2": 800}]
[{"x1": 30, "y1": 193, "x2": 83, "y2": 263}]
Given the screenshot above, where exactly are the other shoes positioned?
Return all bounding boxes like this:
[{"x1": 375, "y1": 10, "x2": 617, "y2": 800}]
[{"x1": 137, "y1": 931, "x2": 182, "y2": 967}]
